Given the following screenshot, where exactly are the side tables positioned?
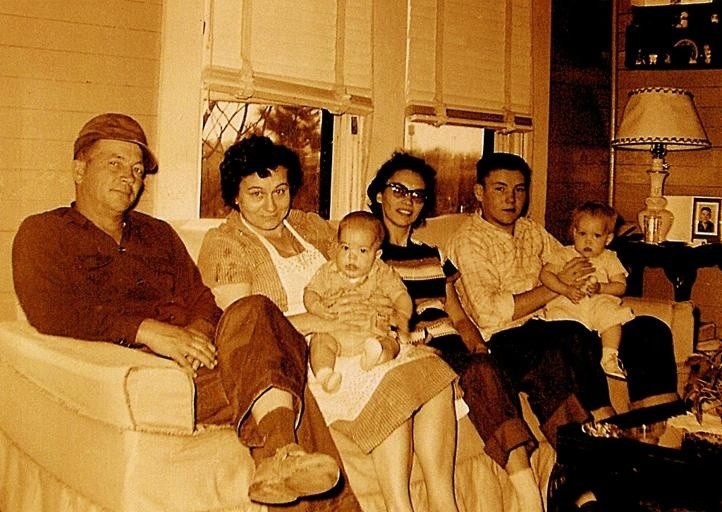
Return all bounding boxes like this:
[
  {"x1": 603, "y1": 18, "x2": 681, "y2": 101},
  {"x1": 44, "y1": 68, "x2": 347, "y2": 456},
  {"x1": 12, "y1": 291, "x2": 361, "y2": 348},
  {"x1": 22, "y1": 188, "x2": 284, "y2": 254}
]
[{"x1": 613, "y1": 241, "x2": 722, "y2": 302}]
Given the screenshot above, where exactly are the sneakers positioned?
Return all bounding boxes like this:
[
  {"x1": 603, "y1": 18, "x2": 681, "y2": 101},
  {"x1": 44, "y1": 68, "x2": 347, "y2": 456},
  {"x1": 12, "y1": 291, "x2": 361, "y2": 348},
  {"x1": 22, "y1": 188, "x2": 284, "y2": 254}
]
[
  {"x1": 600, "y1": 352, "x2": 626, "y2": 380},
  {"x1": 247, "y1": 442, "x2": 342, "y2": 507}
]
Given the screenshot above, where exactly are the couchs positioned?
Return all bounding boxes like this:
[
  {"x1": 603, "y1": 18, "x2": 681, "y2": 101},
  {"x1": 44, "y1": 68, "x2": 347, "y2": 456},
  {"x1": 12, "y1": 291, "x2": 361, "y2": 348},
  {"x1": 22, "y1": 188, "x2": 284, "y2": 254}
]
[{"x1": 0, "y1": 214, "x2": 698, "y2": 512}]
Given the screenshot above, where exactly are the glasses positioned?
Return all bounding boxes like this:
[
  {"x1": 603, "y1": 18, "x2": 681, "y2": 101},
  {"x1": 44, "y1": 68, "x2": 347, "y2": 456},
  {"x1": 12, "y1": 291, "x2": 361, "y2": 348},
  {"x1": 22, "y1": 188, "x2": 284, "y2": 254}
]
[{"x1": 381, "y1": 178, "x2": 429, "y2": 206}]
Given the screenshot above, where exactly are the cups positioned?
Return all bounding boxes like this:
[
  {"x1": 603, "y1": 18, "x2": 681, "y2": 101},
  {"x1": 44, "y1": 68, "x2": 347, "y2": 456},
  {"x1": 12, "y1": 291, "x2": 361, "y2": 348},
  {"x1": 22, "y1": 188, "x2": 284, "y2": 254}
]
[{"x1": 642, "y1": 215, "x2": 663, "y2": 245}]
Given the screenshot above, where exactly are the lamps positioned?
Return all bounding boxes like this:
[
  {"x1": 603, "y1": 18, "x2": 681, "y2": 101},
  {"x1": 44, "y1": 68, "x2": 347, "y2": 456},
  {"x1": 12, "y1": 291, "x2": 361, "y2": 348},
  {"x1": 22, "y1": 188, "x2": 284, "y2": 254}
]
[{"x1": 611, "y1": 87, "x2": 711, "y2": 246}]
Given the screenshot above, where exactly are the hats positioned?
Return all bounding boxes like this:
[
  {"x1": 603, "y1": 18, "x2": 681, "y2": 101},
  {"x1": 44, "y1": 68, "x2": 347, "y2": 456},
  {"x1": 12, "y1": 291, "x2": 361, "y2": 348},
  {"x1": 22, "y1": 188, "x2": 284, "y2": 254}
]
[{"x1": 73, "y1": 111, "x2": 156, "y2": 172}]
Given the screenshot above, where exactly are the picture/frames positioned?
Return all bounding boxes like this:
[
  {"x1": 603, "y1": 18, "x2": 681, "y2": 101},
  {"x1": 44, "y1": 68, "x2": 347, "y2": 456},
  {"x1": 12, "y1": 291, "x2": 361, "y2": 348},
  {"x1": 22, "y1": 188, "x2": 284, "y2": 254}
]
[{"x1": 692, "y1": 196, "x2": 721, "y2": 237}]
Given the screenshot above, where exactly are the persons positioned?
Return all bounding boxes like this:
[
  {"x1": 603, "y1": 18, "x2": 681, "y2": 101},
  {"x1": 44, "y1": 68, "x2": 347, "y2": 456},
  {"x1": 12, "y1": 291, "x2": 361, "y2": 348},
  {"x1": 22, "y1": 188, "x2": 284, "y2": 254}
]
[
  {"x1": 9, "y1": 111, "x2": 363, "y2": 512},
  {"x1": 192, "y1": 133, "x2": 466, "y2": 512},
  {"x1": 536, "y1": 198, "x2": 635, "y2": 385},
  {"x1": 697, "y1": 206, "x2": 714, "y2": 233},
  {"x1": 302, "y1": 210, "x2": 417, "y2": 395},
  {"x1": 448, "y1": 150, "x2": 684, "y2": 423},
  {"x1": 365, "y1": 147, "x2": 545, "y2": 512}
]
